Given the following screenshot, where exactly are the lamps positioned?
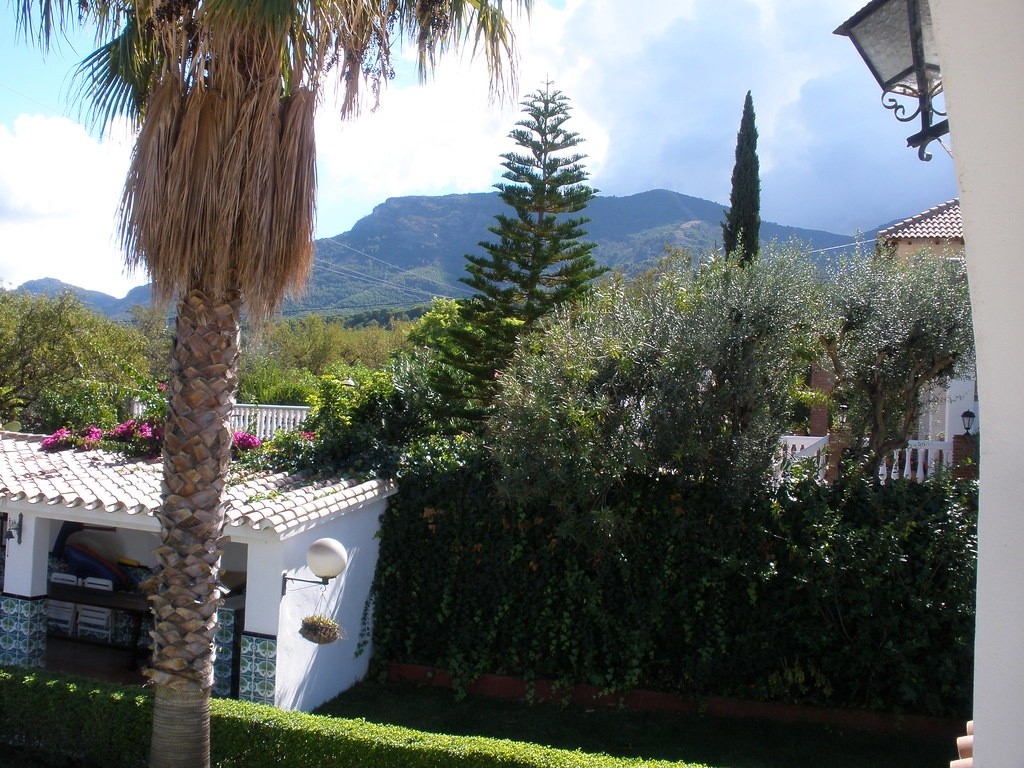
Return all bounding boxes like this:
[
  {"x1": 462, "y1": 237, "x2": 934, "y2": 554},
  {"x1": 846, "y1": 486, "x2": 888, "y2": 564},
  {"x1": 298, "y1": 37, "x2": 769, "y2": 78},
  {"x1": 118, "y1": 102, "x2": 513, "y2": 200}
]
[
  {"x1": 961, "y1": 409, "x2": 976, "y2": 435},
  {"x1": 1, "y1": 513, "x2": 23, "y2": 557},
  {"x1": 838, "y1": 404, "x2": 849, "y2": 426},
  {"x1": 832, "y1": 1, "x2": 950, "y2": 161},
  {"x1": 281, "y1": 538, "x2": 348, "y2": 596}
]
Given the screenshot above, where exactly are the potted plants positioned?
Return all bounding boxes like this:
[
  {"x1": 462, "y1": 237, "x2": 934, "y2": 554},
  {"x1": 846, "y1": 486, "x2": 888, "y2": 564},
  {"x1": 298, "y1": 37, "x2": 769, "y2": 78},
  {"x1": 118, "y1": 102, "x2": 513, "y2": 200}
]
[{"x1": 298, "y1": 613, "x2": 349, "y2": 644}]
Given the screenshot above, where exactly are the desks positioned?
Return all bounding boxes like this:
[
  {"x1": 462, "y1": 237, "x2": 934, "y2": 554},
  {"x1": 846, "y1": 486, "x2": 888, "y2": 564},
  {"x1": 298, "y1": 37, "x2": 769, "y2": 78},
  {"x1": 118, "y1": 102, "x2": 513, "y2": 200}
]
[{"x1": 46, "y1": 581, "x2": 155, "y2": 673}]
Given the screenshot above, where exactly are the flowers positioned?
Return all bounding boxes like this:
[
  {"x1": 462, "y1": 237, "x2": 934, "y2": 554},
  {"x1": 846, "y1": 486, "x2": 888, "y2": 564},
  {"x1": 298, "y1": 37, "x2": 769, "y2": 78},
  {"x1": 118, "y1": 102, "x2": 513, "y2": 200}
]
[{"x1": 40, "y1": 419, "x2": 315, "y2": 462}]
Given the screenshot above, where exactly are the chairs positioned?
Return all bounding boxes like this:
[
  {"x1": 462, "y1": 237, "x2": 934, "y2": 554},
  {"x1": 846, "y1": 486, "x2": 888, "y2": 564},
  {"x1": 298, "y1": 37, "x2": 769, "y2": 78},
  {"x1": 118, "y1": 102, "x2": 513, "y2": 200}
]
[
  {"x1": 46, "y1": 572, "x2": 83, "y2": 636},
  {"x1": 78, "y1": 577, "x2": 114, "y2": 644}
]
